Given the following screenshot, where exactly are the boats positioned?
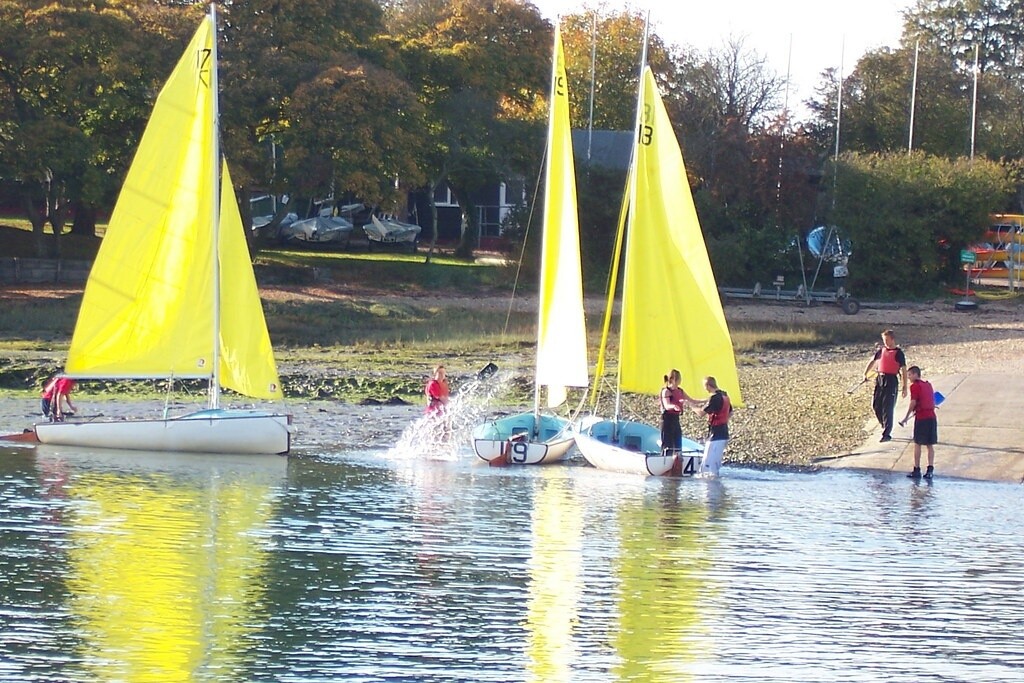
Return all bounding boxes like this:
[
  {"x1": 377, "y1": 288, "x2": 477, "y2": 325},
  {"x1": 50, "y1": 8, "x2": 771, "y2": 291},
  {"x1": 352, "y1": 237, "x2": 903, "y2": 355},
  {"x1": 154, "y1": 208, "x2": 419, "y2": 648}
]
[{"x1": 244, "y1": 172, "x2": 419, "y2": 251}]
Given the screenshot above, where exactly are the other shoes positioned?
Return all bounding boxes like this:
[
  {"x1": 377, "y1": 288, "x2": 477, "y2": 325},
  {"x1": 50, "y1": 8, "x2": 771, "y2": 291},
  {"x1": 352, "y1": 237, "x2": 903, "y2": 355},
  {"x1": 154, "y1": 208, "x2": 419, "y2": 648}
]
[
  {"x1": 923, "y1": 474, "x2": 933, "y2": 479},
  {"x1": 907, "y1": 472, "x2": 921, "y2": 479},
  {"x1": 880, "y1": 435, "x2": 891, "y2": 442}
]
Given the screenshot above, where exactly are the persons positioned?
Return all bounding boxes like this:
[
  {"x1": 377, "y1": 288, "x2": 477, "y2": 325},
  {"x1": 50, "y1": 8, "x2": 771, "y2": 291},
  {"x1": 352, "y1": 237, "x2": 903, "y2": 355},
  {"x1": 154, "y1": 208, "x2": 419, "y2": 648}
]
[
  {"x1": 424, "y1": 365, "x2": 452, "y2": 443},
  {"x1": 864, "y1": 330, "x2": 907, "y2": 442},
  {"x1": 660, "y1": 371, "x2": 734, "y2": 476},
  {"x1": 902, "y1": 366, "x2": 938, "y2": 478},
  {"x1": 42, "y1": 374, "x2": 76, "y2": 420}
]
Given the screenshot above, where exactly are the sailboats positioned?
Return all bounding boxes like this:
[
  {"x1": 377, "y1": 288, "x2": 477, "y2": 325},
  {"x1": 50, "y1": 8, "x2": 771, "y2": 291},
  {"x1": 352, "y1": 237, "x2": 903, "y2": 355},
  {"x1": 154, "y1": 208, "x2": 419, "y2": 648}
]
[
  {"x1": 575, "y1": 6, "x2": 742, "y2": 483},
  {"x1": 0, "y1": 0, "x2": 296, "y2": 456},
  {"x1": 469, "y1": 14, "x2": 589, "y2": 467}
]
